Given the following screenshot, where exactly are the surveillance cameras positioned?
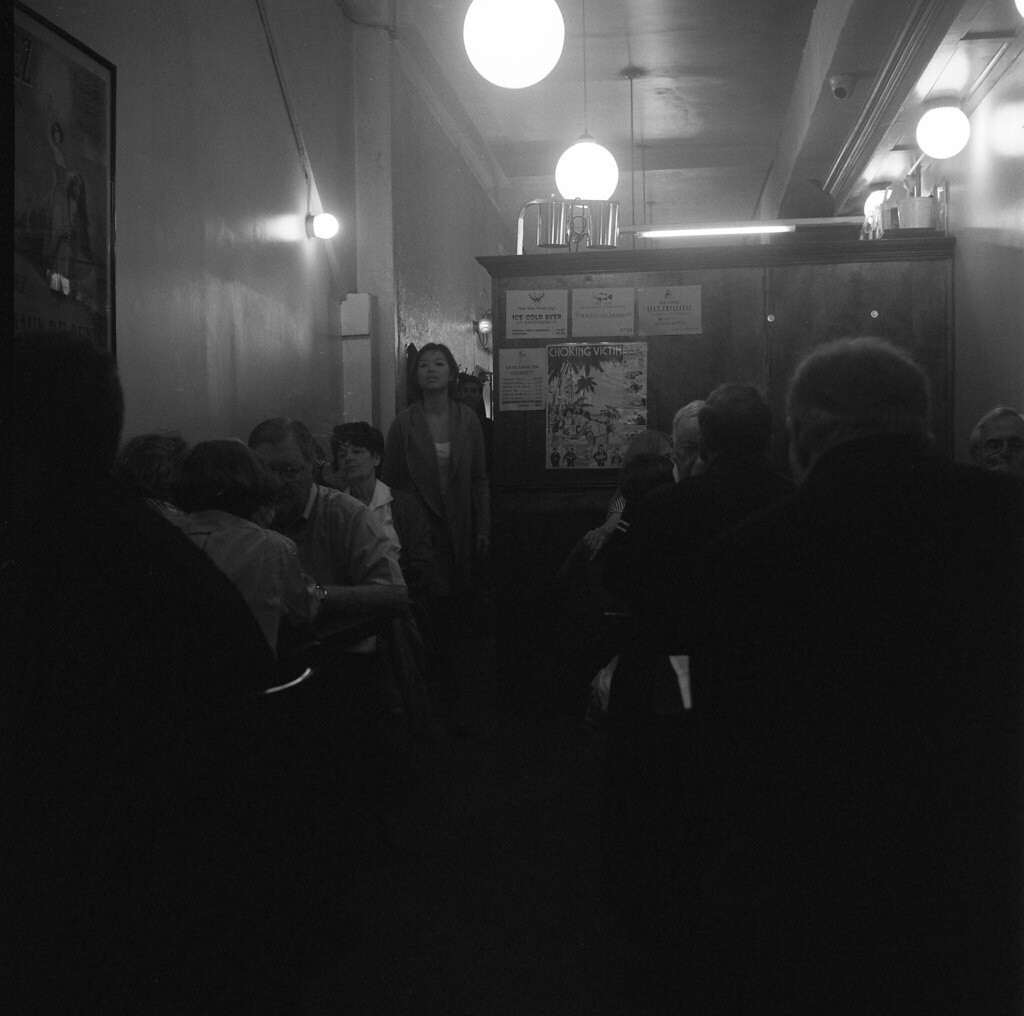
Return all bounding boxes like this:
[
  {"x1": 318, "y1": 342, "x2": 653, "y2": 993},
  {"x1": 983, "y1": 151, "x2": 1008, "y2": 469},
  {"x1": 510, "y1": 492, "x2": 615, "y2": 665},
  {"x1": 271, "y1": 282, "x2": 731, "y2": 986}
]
[{"x1": 830, "y1": 75, "x2": 857, "y2": 102}]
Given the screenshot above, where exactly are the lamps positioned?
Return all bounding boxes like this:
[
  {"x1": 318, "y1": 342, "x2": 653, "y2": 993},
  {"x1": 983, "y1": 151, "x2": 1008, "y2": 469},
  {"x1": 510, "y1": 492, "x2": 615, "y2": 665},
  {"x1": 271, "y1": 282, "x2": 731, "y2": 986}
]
[
  {"x1": 529, "y1": 0, "x2": 618, "y2": 209},
  {"x1": 472, "y1": 319, "x2": 492, "y2": 335},
  {"x1": 915, "y1": 96, "x2": 970, "y2": 159},
  {"x1": 305, "y1": 214, "x2": 339, "y2": 240},
  {"x1": 462, "y1": 0, "x2": 565, "y2": 90}
]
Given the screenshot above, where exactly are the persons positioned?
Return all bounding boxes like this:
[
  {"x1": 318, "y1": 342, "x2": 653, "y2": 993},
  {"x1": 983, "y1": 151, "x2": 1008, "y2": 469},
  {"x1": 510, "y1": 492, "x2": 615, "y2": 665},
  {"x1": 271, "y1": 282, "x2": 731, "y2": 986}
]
[
  {"x1": 12, "y1": 330, "x2": 494, "y2": 1013},
  {"x1": 583, "y1": 340, "x2": 1024, "y2": 1016}
]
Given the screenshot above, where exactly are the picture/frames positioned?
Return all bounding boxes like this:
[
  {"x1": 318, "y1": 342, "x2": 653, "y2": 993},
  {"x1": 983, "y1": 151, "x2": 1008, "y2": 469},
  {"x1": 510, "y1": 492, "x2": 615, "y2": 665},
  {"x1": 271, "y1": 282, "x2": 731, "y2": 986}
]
[{"x1": 0, "y1": 0, "x2": 118, "y2": 363}]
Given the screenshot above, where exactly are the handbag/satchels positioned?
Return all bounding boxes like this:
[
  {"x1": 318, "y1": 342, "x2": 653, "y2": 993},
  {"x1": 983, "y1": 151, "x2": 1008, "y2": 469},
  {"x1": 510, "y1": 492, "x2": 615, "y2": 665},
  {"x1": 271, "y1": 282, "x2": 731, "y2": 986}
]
[{"x1": 584, "y1": 655, "x2": 620, "y2": 741}]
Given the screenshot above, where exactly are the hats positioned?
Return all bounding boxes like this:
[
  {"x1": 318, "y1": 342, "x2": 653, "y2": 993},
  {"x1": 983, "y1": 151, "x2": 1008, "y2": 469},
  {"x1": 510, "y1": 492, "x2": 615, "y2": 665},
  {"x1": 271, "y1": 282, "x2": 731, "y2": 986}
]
[{"x1": 619, "y1": 429, "x2": 675, "y2": 500}]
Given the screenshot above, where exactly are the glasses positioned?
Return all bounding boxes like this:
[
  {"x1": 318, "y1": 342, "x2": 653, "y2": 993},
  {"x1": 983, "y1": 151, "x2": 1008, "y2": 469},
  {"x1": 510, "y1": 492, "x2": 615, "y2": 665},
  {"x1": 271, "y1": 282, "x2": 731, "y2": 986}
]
[{"x1": 278, "y1": 465, "x2": 316, "y2": 480}]
